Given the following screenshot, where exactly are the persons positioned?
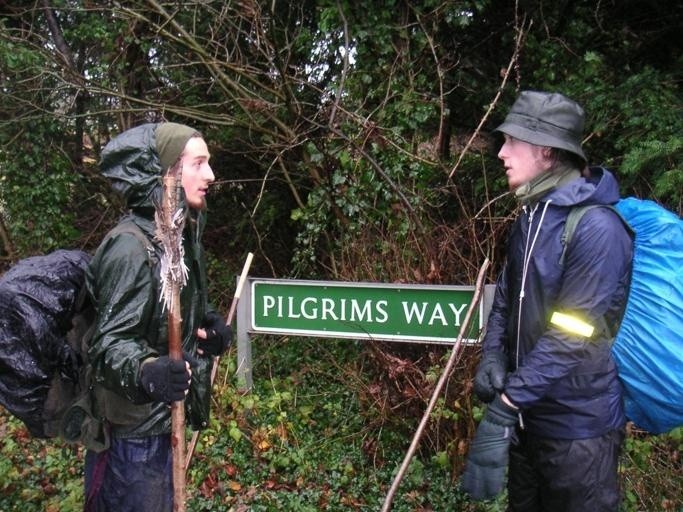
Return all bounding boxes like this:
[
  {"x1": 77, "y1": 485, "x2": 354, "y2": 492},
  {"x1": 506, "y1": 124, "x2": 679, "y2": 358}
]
[
  {"x1": 69, "y1": 123, "x2": 233, "y2": 510},
  {"x1": 457, "y1": 88, "x2": 633, "y2": 509}
]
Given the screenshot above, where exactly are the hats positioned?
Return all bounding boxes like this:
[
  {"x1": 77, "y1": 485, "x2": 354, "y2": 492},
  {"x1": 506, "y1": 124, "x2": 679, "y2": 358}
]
[
  {"x1": 487, "y1": 87, "x2": 591, "y2": 173},
  {"x1": 150, "y1": 120, "x2": 198, "y2": 177}
]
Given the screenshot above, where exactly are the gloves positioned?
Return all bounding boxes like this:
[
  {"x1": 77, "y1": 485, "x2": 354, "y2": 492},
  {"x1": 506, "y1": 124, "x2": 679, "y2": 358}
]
[
  {"x1": 456, "y1": 391, "x2": 523, "y2": 502},
  {"x1": 197, "y1": 310, "x2": 232, "y2": 361},
  {"x1": 137, "y1": 353, "x2": 192, "y2": 405},
  {"x1": 469, "y1": 353, "x2": 509, "y2": 406}
]
